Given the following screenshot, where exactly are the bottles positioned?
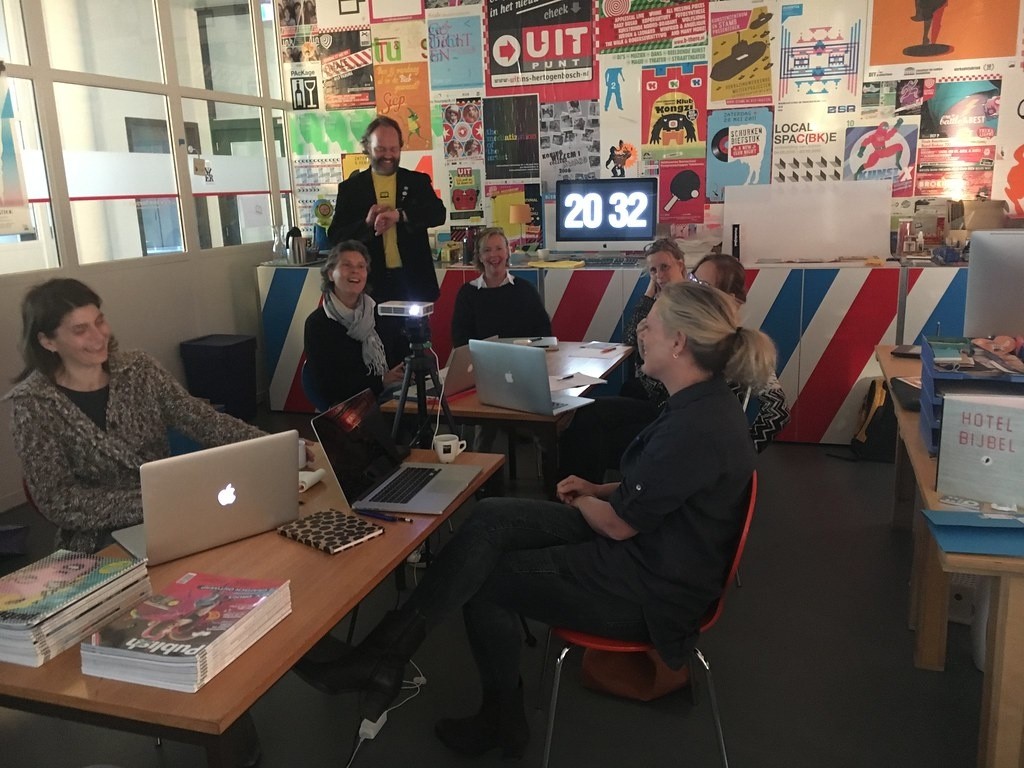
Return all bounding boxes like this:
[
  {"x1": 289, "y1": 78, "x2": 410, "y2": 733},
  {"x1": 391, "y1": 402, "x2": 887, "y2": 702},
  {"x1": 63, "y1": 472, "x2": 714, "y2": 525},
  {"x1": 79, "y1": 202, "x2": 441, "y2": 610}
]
[
  {"x1": 915, "y1": 231, "x2": 924, "y2": 253},
  {"x1": 272, "y1": 224, "x2": 286, "y2": 262}
]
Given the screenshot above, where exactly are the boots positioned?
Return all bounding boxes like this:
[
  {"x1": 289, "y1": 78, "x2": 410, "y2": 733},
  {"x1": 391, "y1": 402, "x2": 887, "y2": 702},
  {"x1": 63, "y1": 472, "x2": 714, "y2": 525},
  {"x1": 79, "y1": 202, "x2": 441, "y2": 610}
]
[
  {"x1": 295, "y1": 611, "x2": 427, "y2": 723},
  {"x1": 435, "y1": 675, "x2": 530, "y2": 763}
]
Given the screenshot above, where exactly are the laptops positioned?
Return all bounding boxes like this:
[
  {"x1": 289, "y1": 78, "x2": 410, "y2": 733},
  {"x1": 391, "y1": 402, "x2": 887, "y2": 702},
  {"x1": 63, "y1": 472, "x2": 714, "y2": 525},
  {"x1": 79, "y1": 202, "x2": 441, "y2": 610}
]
[
  {"x1": 393, "y1": 335, "x2": 499, "y2": 402},
  {"x1": 469, "y1": 340, "x2": 595, "y2": 416},
  {"x1": 311, "y1": 388, "x2": 483, "y2": 513},
  {"x1": 500, "y1": 337, "x2": 558, "y2": 351},
  {"x1": 112, "y1": 430, "x2": 300, "y2": 568}
]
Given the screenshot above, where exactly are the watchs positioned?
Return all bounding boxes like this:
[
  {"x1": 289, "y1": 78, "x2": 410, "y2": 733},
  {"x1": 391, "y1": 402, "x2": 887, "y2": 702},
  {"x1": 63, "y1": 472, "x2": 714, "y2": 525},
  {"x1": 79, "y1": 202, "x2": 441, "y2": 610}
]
[{"x1": 396, "y1": 207, "x2": 404, "y2": 224}]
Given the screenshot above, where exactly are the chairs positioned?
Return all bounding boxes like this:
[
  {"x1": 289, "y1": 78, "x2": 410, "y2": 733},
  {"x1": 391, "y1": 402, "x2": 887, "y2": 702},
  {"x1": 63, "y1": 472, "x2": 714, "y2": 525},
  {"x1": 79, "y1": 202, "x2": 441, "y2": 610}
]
[{"x1": 543, "y1": 466, "x2": 758, "y2": 768}]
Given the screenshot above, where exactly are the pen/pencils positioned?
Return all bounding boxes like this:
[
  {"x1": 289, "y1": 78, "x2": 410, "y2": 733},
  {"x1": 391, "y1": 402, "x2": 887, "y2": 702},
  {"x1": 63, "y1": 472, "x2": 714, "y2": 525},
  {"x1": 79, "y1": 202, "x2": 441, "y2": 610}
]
[
  {"x1": 355, "y1": 507, "x2": 396, "y2": 523},
  {"x1": 558, "y1": 375, "x2": 574, "y2": 381},
  {"x1": 392, "y1": 516, "x2": 414, "y2": 524},
  {"x1": 600, "y1": 346, "x2": 616, "y2": 354},
  {"x1": 528, "y1": 337, "x2": 543, "y2": 343}
]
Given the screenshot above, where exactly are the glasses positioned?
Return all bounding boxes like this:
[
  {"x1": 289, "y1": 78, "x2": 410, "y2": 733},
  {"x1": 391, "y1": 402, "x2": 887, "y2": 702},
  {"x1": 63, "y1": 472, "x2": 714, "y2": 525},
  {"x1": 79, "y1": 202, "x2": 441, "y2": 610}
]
[
  {"x1": 643, "y1": 238, "x2": 676, "y2": 253},
  {"x1": 688, "y1": 273, "x2": 710, "y2": 286}
]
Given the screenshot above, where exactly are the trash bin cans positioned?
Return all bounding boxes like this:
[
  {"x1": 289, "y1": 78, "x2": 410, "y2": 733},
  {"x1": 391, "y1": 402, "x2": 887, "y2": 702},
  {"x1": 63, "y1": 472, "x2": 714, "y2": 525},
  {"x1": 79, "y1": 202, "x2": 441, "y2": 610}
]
[{"x1": 179, "y1": 334, "x2": 259, "y2": 420}]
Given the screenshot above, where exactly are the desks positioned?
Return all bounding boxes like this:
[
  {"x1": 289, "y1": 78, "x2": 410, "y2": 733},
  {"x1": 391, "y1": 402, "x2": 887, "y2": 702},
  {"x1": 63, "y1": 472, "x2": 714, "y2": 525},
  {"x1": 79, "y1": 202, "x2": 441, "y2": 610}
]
[
  {"x1": 381, "y1": 336, "x2": 635, "y2": 497},
  {"x1": 0, "y1": 442, "x2": 504, "y2": 768},
  {"x1": 876, "y1": 346, "x2": 1024, "y2": 768}
]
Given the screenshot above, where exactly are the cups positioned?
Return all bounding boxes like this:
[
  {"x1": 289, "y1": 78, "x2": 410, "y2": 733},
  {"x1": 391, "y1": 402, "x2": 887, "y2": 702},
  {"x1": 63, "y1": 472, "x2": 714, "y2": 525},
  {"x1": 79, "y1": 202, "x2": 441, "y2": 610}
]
[
  {"x1": 298, "y1": 440, "x2": 307, "y2": 470},
  {"x1": 433, "y1": 433, "x2": 466, "y2": 465}
]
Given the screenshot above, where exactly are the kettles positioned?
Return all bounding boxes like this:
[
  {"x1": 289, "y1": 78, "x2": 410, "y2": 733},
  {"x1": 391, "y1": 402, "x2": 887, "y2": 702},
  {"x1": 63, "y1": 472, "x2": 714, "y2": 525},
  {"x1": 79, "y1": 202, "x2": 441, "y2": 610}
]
[{"x1": 285, "y1": 227, "x2": 306, "y2": 265}]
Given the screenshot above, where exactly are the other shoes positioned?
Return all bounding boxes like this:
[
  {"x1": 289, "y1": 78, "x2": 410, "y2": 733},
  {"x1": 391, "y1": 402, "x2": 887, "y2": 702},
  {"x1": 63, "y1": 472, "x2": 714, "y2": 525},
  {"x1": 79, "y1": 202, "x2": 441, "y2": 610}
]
[{"x1": 407, "y1": 541, "x2": 434, "y2": 568}]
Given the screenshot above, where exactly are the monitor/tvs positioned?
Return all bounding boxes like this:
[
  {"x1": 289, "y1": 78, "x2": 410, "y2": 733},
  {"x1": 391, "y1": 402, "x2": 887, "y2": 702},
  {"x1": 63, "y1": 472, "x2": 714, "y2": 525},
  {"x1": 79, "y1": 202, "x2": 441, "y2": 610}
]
[
  {"x1": 554, "y1": 175, "x2": 660, "y2": 257},
  {"x1": 962, "y1": 230, "x2": 1024, "y2": 337}
]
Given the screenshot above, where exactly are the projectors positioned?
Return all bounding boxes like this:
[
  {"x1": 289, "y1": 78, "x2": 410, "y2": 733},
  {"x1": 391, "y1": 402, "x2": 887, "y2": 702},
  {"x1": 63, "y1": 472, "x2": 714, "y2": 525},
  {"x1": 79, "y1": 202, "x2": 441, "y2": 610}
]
[{"x1": 378, "y1": 301, "x2": 434, "y2": 317}]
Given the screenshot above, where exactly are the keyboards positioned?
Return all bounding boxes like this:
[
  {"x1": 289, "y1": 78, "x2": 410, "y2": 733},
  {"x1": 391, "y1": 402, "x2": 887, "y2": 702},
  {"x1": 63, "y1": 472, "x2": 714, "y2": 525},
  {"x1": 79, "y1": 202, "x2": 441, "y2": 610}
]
[{"x1": 552, "y1": 258, "x2": 638, "y2": 265}]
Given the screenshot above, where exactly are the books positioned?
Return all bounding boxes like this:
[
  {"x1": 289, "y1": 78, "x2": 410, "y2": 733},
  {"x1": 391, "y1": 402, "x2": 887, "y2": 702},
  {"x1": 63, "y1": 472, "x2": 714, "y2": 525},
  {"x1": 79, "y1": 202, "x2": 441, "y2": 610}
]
[
  {"x1": 0, "y1": 548, "x2": 155, "y2": 666},
  {"x1": 78, "y1": 569, "x2": 293, "y2": 693},
  {"x1": 298, "y1": 469, "x2": 325, "y2": 492},
  {"x1": 278, "y1": 508, "x2": 384, "y2": 553}
]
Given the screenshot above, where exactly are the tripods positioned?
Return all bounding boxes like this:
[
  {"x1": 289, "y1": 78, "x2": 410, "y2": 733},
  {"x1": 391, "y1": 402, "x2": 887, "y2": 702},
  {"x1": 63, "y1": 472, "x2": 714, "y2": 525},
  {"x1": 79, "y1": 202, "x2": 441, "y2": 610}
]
[{"x1": 390, "y1": 319, "x2": 481, "y2": 503}]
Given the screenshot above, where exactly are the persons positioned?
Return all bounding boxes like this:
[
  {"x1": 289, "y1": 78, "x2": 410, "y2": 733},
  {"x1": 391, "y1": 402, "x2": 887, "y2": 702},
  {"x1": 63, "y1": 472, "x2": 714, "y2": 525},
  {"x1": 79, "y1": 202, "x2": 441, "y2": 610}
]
[
  {"x1": 623, "y1": 238, "x2": 687, "y2": 397},
  {"x1": 1, "y1": 278, "x2": 316, "y2": 554},
  {"x1": 452, "y1": 228, "x2": 557, "y2": 470},
  {"x1": 305, "y1": 240, "x2": 437, "y2": 449},
  {"x1": 328, "y1": 115, "x2": 446, "y2": 369},
  {"x1": 291, "y1": 281, "x2": 785, "y2": 763},
  {"x1": 559, "y1": 254, "x2": 798, "y2": 501}
]
